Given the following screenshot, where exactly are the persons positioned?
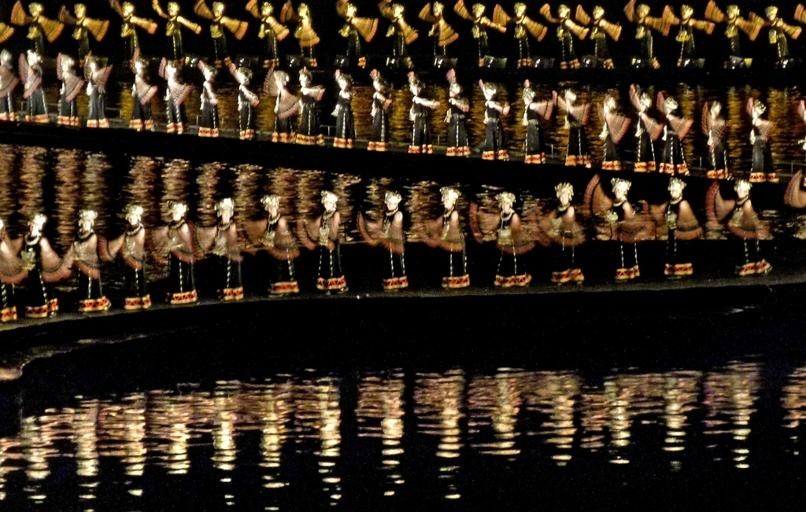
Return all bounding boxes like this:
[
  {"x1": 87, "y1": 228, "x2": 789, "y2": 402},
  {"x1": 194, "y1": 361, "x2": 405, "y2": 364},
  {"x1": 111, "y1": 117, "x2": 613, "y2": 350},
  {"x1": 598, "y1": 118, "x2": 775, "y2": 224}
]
[{"x1": 0, "y1": 0, "x2": 806, "y2": 329}]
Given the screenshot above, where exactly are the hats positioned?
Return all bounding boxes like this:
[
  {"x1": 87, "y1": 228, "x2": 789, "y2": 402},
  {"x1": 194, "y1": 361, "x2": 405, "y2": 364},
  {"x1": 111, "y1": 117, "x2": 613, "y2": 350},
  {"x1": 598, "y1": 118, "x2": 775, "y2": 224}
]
[
  {"x1": 28, "y1": 177, "x2": 751, "y2": 231},
  {"x1": 0, "y1": 50, "x2": 769, "y2": 119},
  {"x1": 27, "y1": 1, "x2": 782, "y2": 23}
]
